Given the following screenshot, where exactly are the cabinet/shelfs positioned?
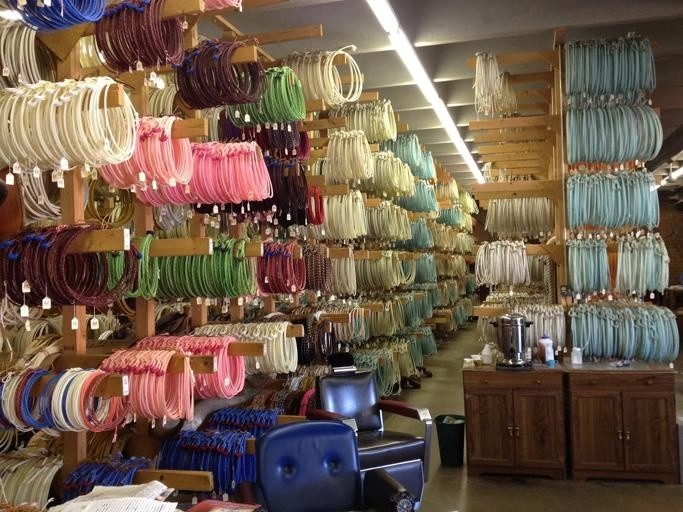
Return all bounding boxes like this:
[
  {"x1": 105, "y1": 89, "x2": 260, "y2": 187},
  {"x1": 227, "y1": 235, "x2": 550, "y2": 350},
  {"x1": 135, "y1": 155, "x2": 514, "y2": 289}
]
[
  {"x1": 558, "y1": 351, "x2": 680, "y2": 486},
  {"x1": 457, "y1": 352, "x2": 570, "y2": 483}
]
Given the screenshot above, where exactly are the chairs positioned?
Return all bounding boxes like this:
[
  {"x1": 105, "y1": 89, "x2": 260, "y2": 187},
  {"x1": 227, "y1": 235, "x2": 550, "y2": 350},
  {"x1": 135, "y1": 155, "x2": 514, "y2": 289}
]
[
  {"x1": 307, "y1": 371, "x2": 435, "y2": 512},
  {"x1": 254, "y1": 415, "x2": 414, "y2": 511}
]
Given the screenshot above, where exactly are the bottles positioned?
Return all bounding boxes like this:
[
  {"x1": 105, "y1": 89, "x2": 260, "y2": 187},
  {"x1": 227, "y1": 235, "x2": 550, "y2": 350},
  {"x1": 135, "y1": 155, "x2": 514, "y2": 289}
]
[
  {"x1": 537, "y1": 333, "x2": 554, "y2": 362},
  {"x1": 525, "y1": 342, "x2": 583, "y2": 364},
  {"x1": 482, "y1": 344, "x2": 492, "y2": 365}
]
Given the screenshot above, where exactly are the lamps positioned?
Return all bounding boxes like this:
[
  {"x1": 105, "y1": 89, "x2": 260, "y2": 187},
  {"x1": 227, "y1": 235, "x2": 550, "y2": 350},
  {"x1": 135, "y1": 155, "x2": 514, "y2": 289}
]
[{"x1": 362, "y1": 0, "x2": 486, "y2": 189}]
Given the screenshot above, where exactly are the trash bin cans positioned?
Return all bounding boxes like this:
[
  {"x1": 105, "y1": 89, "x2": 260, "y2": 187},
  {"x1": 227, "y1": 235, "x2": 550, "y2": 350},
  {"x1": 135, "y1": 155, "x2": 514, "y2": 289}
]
[{"x1": 434, "y1": 414, "x2": 465, "y2": 468}]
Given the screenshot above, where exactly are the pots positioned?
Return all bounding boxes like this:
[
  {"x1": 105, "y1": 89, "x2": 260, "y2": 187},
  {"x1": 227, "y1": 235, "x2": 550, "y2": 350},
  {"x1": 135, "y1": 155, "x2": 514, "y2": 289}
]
[{"x1": 488, "y1": 312, "x2": 534, "y2": 370}]
